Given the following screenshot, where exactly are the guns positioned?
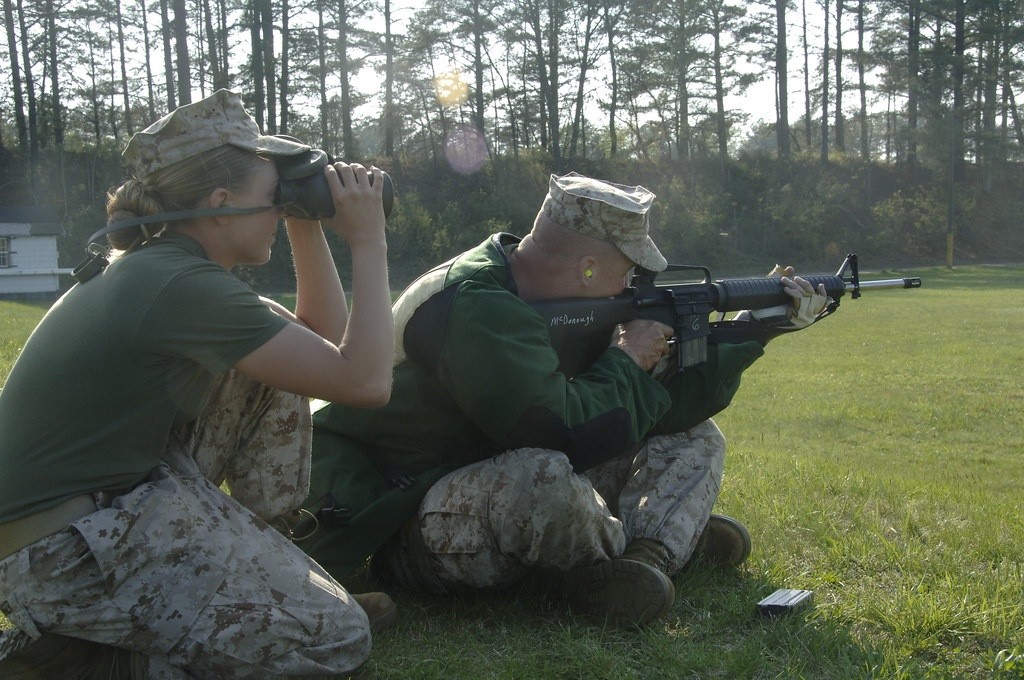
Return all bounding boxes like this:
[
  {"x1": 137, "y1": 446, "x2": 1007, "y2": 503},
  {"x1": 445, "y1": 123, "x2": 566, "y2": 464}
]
[{"x1": 535, "y1": 250, "x2": 923, "y2": 367}]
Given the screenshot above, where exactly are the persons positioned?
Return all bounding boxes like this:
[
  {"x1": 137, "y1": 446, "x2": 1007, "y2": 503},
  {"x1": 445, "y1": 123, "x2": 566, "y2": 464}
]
[
  {"x1": 294, "y1": 172, "x2": 832, "y2": 631},
  {"x1": 1, "y1": 88, "x2": 395, "y2": 680}
]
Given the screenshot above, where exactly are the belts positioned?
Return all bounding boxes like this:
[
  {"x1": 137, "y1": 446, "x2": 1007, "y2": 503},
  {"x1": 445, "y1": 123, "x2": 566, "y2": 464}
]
[{"x1": 0, "y1": 490, "x2": 113, "y2": 562}]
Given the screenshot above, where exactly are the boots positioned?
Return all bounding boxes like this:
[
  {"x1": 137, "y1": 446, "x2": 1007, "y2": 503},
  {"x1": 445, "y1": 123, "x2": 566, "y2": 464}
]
[
  {"x1": 0, "y1": 623, "x2": 130, "y2": 680},
  {"x1": 688, "y1": 514, "x2": 753, "y2": 575},
  {"x1": 263, "y1": 510, "x2": 401, "y2": 635},
  {"x1": 564, "y1": 538, "x2": 679, "y2": 626}
]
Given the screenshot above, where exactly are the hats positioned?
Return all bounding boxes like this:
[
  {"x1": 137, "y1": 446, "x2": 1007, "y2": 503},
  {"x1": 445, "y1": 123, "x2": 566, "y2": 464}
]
[
  {"x1": 121, "y1": 89, "x2": 312, "y2": 179},
  {"x1": 540, "y1": 171, "x2": 667, "y2": 273}
]
